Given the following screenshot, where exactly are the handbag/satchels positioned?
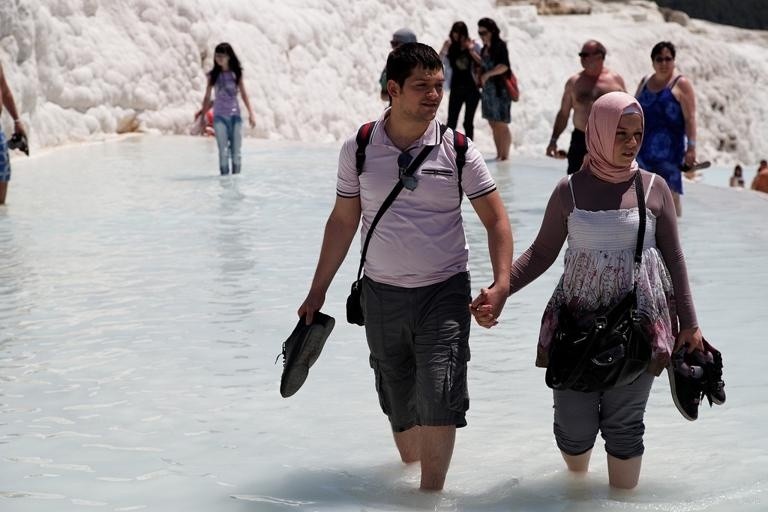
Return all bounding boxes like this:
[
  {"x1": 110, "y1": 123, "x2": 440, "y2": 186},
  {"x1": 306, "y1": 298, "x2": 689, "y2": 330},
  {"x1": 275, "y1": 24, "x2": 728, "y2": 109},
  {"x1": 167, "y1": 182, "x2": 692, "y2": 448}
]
[
  {"x1": 545, "y1": 299, "x2": 653, "y2": 393},
  {"x1": 346, "y1": 276, "x2": 368, "y2": 326},
  {"x1": 505, "y1": 73, "x2": 522, "y2": 103}
]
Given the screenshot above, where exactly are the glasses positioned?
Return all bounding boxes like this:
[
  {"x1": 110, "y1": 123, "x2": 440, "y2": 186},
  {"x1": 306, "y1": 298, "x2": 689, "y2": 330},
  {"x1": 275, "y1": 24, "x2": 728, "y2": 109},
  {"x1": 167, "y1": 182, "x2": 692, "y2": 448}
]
[
  {"x1": 476, "y1": 29, "x2": 490, "y2": 36},
  {"x1": 654, "y1": 56, "x2": 674, "y2": 62}
]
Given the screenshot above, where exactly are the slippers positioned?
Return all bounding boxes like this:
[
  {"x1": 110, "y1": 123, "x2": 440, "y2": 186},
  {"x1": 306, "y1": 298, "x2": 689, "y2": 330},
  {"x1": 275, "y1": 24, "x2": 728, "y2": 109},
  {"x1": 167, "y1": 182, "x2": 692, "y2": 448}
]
[
  {"x1": 679, "y1": 159, "x2": 712, "y2": 173},
  {"x1": 6, "y1": 132, "x2": 31, "y2": 157},
  {"x1": 544, "y1": 148, "x2": 568, "y2": 159}
]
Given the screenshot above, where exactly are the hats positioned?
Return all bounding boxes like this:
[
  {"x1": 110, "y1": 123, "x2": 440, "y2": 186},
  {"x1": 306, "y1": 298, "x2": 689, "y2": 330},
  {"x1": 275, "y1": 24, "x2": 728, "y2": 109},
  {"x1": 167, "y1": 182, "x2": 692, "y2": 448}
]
[{"x1": 389, "y1": 26, "x2": 418, "y2": 44}]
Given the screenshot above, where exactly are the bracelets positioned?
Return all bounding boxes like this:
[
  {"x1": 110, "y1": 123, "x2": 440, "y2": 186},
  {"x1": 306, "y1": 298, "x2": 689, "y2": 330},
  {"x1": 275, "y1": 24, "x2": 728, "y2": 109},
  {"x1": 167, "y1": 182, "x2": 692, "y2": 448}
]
[{"x1": 15, "y1": 118, "x2": 20, "y2": 124}]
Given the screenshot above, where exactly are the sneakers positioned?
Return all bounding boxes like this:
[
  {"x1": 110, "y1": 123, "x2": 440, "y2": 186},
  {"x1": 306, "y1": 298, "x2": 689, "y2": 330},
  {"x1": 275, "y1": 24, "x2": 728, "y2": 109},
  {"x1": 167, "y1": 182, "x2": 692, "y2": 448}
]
[
  {"x1": 309, "y1": 309, "x2": 335, "y2": 373},
  {"x1": 692, "y1": 336, "x2": 727, "y2": 406},
  {"x1": 280, "y1": 312, "x2": 326, "y2": 400},
  {"x1": 667, "y1": 349, "x2": 702, "y2": 422}
]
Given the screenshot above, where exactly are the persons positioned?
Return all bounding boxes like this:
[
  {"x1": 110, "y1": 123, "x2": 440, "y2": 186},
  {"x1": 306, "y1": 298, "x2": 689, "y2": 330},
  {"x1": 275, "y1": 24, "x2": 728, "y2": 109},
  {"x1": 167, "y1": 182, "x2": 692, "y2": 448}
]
[
  {"x1": 477, "y1": 90, "x2": 704, "y2": 489},
  {"x1": 730, "y1": 165, "x2": 745, "y2": 188},
  {"x1": 297, "y1": 41, "x2": 513, "y2": 493},
  {"x1": 196, "y1": 43, "x2": 255, "y2": 175},
  {"x1": 750, "y1": 161, "x2": 767, "y2": 191},
  {"x1": 0, "y1": 61, "x2": 26, "y2": 205}
]
